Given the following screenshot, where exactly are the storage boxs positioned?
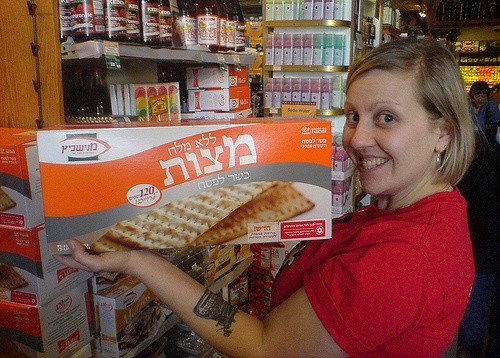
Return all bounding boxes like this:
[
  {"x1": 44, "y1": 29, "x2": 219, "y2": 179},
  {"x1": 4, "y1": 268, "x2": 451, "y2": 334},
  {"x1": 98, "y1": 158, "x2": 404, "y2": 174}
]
[
  {"x1": 110, "y1": 82, "x2": 180, "y2": 121},
  {"x1": 195, "y1": 111, "x2": 214, "y2": 120},
  {"x1": 215, "y1": 108, "x2": 252, "y2": 120},
  {"x1": 93, "y1": 275, "x2": 166, "y2": 358},
  {"x1": 36, "y1": 120, "x2": 333, "y2": 255},
  {"x1": 0, "y1": 281, "x2": 97, "y2": 358},
  {"x1": 0, "y1": 127, "x2": 45, "y2": 228},
  {"x1": 186, "y1": 65, "x2": 245, "y2": 90},
  {"x1": 0, "y1": 222, "x2": 94, "y2": 308},
  {"x1": 188, "y1": 86, "x2": 250, "y2": 112}
]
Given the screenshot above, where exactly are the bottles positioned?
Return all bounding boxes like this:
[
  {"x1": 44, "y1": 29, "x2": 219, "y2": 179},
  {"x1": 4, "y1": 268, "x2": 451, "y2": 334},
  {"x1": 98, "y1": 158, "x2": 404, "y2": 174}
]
[
  {"x1": 382, "y1": 1, "x2": 396, "y2": 27},
  {"x1": 57, "y1": 0, "x2": 262, "y2": 78},
  {"x1": 63, "y1": 65, "x2": 110, "y2": 116},
  {"x1": 436, "y1": 0, "x2": 500, "y2": 21},
  {"x1": 363, "y1": 16, "x2": 375, "y2": 51},
  {"x1": 229, "y1": 267, "x2": 252, "y2": 307},
  {"x1": 332, "y1": 145, "x2": 351, "y2": 207}
]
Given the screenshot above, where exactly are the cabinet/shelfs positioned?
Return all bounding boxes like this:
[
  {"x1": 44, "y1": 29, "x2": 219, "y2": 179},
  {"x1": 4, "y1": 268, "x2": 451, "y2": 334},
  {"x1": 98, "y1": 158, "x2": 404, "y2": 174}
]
[{"x1": 262, "y1": 0, "x2": 358, "y2": 208}]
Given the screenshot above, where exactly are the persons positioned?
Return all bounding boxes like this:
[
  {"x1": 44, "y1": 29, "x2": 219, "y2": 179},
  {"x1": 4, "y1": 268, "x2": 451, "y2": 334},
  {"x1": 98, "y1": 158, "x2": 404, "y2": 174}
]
[
  {"x1": 54, "y1": 38, "x2": 476, "y2": 358},
  {"x1": 467, "y1": 81, "x2": 500, "y2": 161}
]
